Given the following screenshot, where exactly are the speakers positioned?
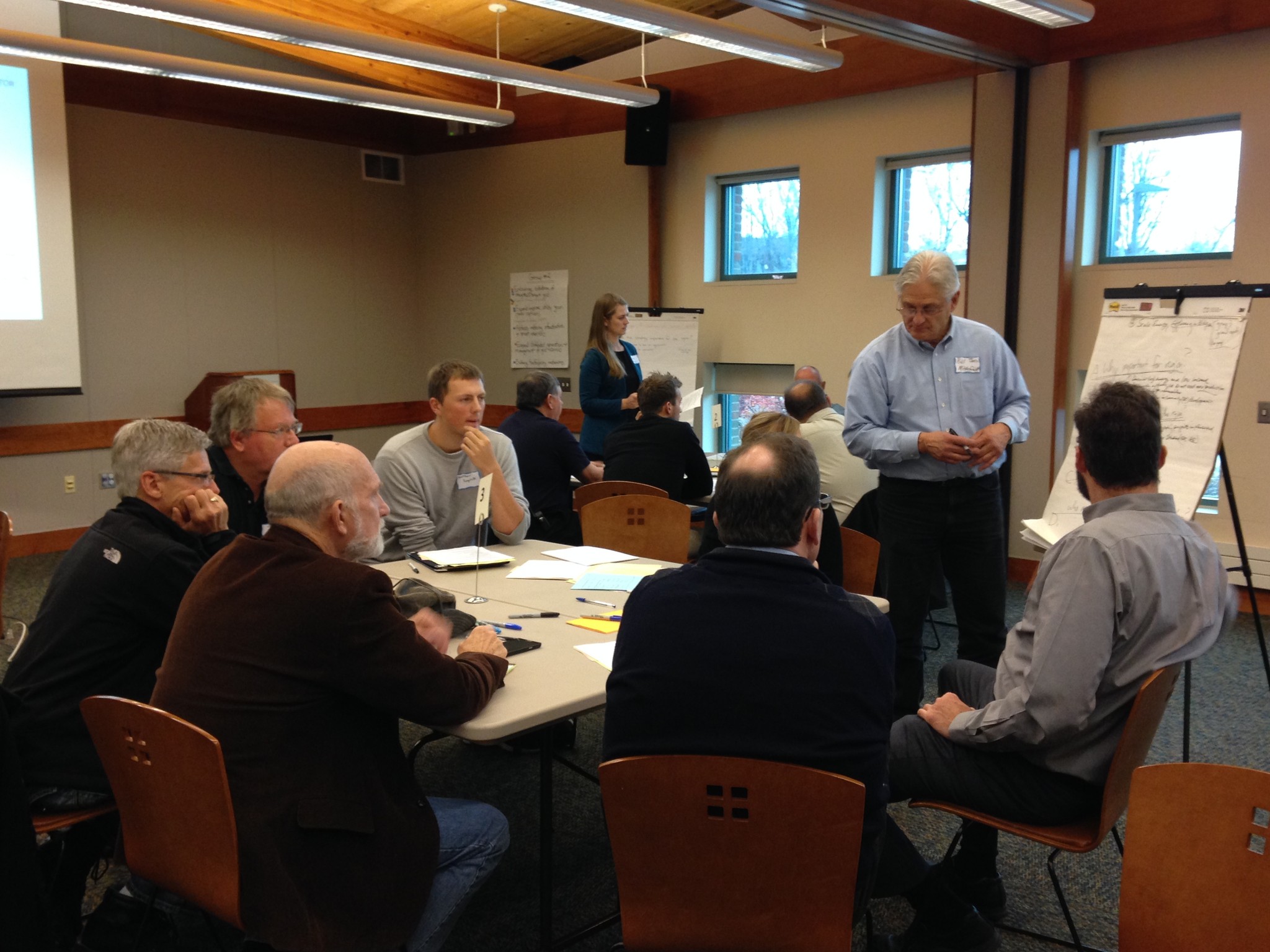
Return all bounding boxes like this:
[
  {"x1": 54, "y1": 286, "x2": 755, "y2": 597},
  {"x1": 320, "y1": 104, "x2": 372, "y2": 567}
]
[{"x1": 621, "y1": 82, "x2": 669, "y2": 167}]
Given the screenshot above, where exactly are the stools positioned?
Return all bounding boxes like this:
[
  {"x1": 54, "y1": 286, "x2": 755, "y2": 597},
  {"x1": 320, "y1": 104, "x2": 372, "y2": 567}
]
[{"x1": 16, "y1": 796, "x2": 124, "y2": 951}]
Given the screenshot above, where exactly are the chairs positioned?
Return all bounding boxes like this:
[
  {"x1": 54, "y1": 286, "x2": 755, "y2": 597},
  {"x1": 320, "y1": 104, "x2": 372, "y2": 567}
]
[
  {"x1": 571, "y1": 479, "x2": 668, "y2": 523},
  {"x1": 910, "y1": 649, "x2": 1188, "y2": 952},
  {"x1": 591, "y1": 753, "x2": 867, "y2": 952},
  {"x1": 580, "y1": 495, "x2": 692, "y2": 564},
  {"x1": 1113, "y1": 763, "x2": 1269, "y2": 952},
  {"x1": 75, "y1": 695, "x2": 257, "y2": 951},
  {"x1": 831, "y1": 529, "x2": 884, "y2": 595}
]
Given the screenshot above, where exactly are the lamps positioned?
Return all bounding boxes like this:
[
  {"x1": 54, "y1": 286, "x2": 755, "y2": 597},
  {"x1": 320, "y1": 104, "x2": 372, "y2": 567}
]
[{"x1": 0, "y1": 1, "x2": 1100, "y2": 129}]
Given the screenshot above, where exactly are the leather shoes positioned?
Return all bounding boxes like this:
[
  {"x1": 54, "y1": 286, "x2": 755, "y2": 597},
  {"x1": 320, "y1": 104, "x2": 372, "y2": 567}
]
[
  {"x1": 870, "y1": 905, "x2": 1003, "y2": 952},
  {"x1": 929, "y1": 854, "x2": 1006, "y2": 909}
]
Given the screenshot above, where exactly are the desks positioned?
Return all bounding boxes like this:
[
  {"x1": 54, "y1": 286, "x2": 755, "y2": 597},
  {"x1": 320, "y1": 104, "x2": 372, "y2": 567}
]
[{"x1": 348, "y1": 533, "x2": 894, "y2": 931}]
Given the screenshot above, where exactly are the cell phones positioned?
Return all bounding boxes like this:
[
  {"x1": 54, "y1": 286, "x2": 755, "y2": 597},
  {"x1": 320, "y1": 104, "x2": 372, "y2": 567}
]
[{"x1": 503, "y1": 638, "x2": 545, "y2": 658}]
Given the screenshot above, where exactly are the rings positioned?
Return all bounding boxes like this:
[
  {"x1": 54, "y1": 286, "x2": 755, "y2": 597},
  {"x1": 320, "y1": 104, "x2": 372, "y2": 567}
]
[{"x1": 209, "y1": 497, "x2": 220, "y2": 504}]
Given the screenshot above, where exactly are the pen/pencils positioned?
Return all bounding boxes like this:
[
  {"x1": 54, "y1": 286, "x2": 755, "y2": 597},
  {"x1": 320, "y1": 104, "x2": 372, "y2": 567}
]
[
  {"x1": 949, "y1": 427, "x2": 973, "y2": 456},
  {"x1": 508, "y1": 612, "x2": 561, "y2": 620},
  {"x1": 580, "y1": 614, "x2": 622, "y2": 622},
  {"x1": 409, "y1": 562, "x2": 419, "y2": 573},
  {"x1": 575, "y1": 597, "x2": 616, "y2": 608},
  {"x1": 482, "y1": 621, "x2": 522, "y2": 631},
  {"x1": 478, "y1": 621, "x2": 501, "y2": 634}
]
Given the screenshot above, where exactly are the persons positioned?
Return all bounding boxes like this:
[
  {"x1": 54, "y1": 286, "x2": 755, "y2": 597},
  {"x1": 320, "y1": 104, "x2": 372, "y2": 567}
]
[
  {"x1": 499, "y1": 370, "x2": 605, "y2": 543},
  {"x1": 868, "y1": 380, "x2": 1229, "y2": 952},
  {"x1": 147, "y1": 438, "x2": 512, "y2": 951},
  {"x1": 795, "y1": 365, "x2": 846, "y2": 415},
  {"x1": 784, "y1": 378, "x2": 880, "y2": 525},
  {"x1": 369, "y1": 358, "x2": 532, "y2": 565},
  {"x1": 577, "y1": 292, "x2": 646, "y2": 466},
  {"x1": 840, "y1": 255, "x2": 1032, "y2": 721},
  {"x1": 1, "y1": 416, "x2": 236, "y2": 950},
  {"x1": 203, "y1": 376, "x2": 304, "y2": 540},
  {"x1": 604, "y1": 432, "x2": 898, "y2": 937},
  {"x1": 602, "y1": 373, "x2": 713, "y2": 562},
  {"x1": 692, "y1": 410, "x2": 849, "y2": 586}
]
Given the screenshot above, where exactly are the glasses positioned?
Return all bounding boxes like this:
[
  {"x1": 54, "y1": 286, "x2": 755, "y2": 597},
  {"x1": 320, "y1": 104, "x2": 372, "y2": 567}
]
[
  {"x1": 895, "y1": 296, "x2": 953, "y2": 316},
  {"x1": 242, "y1": 422, "x2": 303, "y2": 438},
  {"x1": 151, "y1": 470, "x2": 215, "y2": 487}
]
